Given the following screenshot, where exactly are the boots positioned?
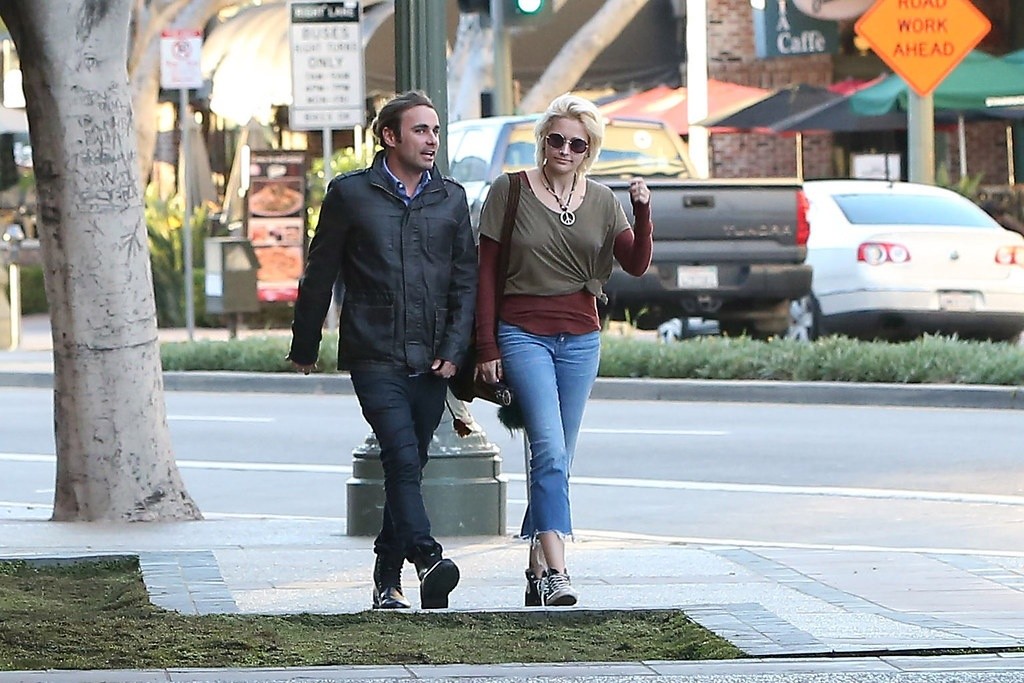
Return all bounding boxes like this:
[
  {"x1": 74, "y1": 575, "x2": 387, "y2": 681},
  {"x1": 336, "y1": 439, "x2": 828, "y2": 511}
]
[
  {"x1": 371, "y1": 553, "x2": 411, "y2": 610},
  {"x1": 416, "y1": 549, "x2": 460, "y2": 609}
]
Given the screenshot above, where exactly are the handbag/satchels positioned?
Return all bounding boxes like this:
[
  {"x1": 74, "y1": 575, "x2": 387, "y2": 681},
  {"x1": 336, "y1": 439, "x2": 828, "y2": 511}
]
[{"x1": 448, "y1": 342, "x2": 512, "y2": 409}]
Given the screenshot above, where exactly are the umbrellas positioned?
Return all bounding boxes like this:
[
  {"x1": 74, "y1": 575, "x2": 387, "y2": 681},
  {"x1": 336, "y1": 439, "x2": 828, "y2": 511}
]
[{"x1": 576, "y1": 51, "x2": 1024, "y2": 185}]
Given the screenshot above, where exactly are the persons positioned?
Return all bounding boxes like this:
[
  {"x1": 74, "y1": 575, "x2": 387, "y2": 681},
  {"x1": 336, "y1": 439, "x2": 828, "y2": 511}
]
[
  {"x1": 476, "y1": 92, "x2": 653, "y2": 606},
  {"x1": 284, "y1": 88, "x2": 477, "y2": 610}
]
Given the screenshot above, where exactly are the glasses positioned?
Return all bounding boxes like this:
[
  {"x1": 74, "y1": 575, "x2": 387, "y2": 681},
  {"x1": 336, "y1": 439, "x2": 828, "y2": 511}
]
[{"x1": 543, "y1": 132, "x2": 590, "y2": 155}]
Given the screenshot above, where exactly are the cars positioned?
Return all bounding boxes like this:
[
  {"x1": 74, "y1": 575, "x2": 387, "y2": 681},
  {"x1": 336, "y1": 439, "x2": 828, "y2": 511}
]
[{"x1": 790, "y1": 178, "x2": 1024, "y2": 346}]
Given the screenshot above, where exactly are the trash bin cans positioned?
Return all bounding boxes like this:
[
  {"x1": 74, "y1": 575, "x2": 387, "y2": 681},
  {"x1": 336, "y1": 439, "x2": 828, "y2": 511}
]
[{"x1": 203, "y1": 235, "x2": 263, "y2": 315}]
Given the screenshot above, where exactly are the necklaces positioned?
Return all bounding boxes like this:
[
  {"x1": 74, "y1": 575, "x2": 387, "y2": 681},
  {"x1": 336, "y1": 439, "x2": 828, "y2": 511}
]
[{"x1": 540, "y1": 166, "x2": 577, "y2": 225}]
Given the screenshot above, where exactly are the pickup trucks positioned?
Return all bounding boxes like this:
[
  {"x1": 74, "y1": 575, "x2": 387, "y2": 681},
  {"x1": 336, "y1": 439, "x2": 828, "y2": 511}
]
[{"x1": 414, "y1": 113, "x2": 816, "y2": 342}]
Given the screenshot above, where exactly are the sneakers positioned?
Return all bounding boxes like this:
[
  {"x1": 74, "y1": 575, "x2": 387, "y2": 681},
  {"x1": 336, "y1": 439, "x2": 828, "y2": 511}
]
[
  {"x1": 542, "y1": 567, "x2": 581, "y2": 607},
  {"x1": 524, "y1": 569, "x2": 546, "y2": 606}
]
